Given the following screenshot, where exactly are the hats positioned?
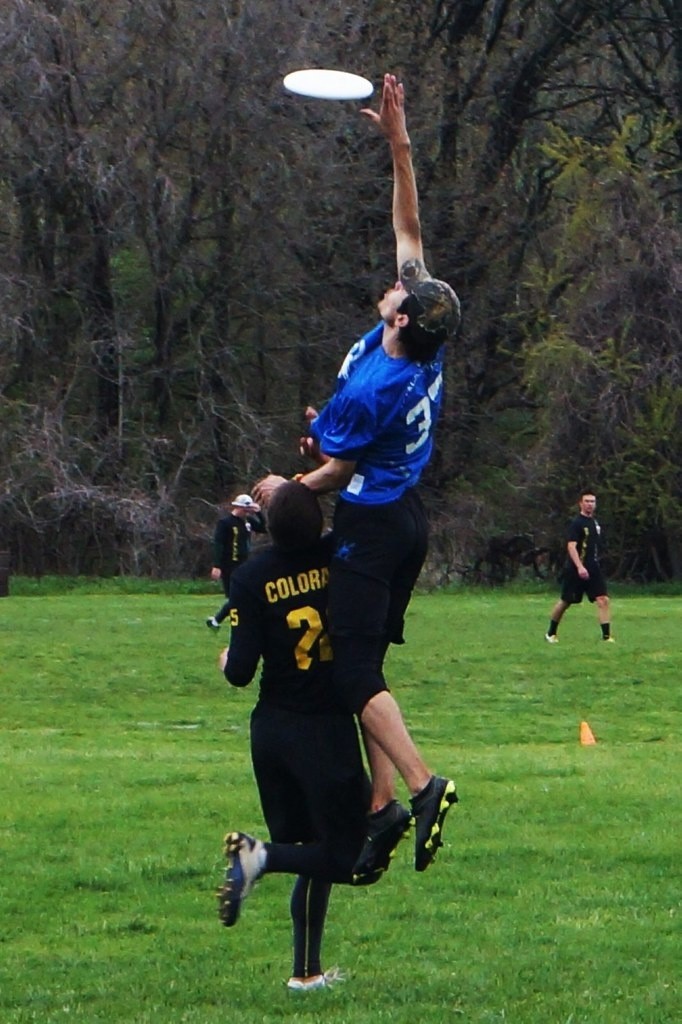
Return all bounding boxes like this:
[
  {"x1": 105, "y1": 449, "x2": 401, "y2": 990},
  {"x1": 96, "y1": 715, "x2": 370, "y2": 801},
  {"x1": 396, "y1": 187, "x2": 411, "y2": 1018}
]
[
  {"x1": 231, "y1": 494, "x2": 253, "y2": 507},
  {"x1": 400, "y1": 258, "x2": 462, "y2": 337}
]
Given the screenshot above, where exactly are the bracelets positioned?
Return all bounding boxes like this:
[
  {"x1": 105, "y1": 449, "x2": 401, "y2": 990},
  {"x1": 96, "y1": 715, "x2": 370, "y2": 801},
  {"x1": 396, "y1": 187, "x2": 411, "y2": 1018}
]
[{"x1": 291, "y1": 473, "x2": 304, "y2": 483}]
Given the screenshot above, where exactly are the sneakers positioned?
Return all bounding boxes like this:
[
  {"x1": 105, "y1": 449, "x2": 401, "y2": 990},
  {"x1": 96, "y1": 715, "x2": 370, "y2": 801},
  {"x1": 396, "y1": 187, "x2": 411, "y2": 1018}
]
[
  {"x1": 410, "y1": 774, "x2": 459, "y2": 871},
  {"x1": 205, "y1": 615, "x2": 220, "y2": 632},
  {"x1": 216, "y1": 831, "x2": 264, "y2": 928},
  {"x1": 544, "y1": 633, "x2": 559, "y2": 643},
  {"x1": 288, "y1": 973, "x2": 346, "y2": 989},
  {"x1": 600, "y1": 636, "x2": 615, "y2": 643},
  {"x1": 352, "y1": 799, "x2": 411, "y2": 886}
]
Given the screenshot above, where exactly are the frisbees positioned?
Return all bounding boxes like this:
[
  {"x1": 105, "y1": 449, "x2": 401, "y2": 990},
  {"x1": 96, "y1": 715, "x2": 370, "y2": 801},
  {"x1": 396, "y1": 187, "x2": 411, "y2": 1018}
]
[{"x1": 283, "y1": 68, "x2": 376, "y2": 100}]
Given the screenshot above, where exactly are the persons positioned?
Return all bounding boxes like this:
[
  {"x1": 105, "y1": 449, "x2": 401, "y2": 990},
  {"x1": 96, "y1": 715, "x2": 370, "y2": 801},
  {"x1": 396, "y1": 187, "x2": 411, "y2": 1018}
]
[
  {"x1": 206, "y1": 494, "x2": 260, "y2": 636},
  {"x1": 250, "y1": 73, "x2": 462, "y2": 885},
  {"x1": 544, "y1": 489, "x2": 618, "y2": 643},
  {"x1": 212, "y1": 406, "x2": 384, "y2": 992}
]
